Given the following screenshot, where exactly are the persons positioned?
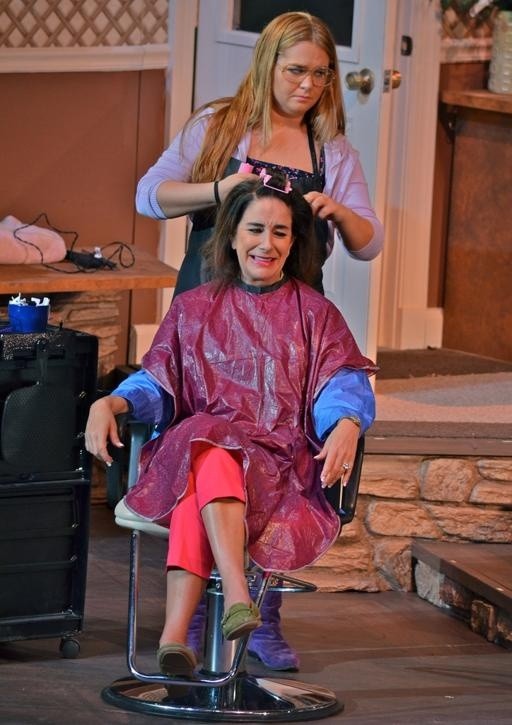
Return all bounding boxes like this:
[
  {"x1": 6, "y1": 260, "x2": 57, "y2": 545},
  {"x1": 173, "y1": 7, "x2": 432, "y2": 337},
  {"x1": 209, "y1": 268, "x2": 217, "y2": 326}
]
[
  {"x1": 84, "y1": 174, "x2": 379, "y2": 700},
  {"x1": 135, "y1": 11, "x2": 383, "y2": 672}
]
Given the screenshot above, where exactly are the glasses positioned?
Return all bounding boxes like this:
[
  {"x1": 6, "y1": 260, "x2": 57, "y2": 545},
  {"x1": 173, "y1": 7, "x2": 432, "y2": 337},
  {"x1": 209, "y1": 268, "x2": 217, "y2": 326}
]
[{"x1": 272, "y1": 60, "x2": 337, "y2": 90}]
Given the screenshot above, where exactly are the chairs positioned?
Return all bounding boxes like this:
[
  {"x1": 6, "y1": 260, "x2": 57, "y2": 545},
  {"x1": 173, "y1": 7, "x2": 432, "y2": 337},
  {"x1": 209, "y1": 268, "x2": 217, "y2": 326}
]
[{"x1": 100, "y1": 414, "x2": 365, "y2": 722}]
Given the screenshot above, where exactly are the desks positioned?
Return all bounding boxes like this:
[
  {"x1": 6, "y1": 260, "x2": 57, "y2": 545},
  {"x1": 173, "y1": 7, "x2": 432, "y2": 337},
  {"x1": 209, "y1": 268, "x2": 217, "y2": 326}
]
[{"x1": 0, "y1": 246, "x2": 179, "y2": 366}]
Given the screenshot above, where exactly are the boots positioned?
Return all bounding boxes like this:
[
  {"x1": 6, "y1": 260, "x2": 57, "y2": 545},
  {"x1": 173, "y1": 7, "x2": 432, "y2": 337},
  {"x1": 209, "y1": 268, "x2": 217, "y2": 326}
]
[{"x1": 247, "y1": 579, "x2": 300, "y2": 677}]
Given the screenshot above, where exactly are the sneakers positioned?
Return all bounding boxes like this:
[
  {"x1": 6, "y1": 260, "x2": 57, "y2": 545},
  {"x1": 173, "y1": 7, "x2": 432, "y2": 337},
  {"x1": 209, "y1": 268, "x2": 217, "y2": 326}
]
[
  {"x1": 158, "y1": 645, "x2": 194, "y2": 697},
  {"x1": 221, "y1": 601, "x2": 262, "y2": 638}
]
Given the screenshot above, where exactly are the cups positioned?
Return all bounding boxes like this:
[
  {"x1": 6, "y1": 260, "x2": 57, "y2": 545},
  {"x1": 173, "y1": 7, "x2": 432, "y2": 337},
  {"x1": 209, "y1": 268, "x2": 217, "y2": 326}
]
[{"x1": 8, "y1": 303, "x2": 51, "y2": 333}]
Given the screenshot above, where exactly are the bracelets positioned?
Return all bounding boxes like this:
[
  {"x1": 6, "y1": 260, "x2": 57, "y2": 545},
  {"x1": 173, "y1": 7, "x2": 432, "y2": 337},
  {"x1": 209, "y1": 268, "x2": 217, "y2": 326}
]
[{"x1": 214, "y1": 180, "x2": 221, "y2": 206}]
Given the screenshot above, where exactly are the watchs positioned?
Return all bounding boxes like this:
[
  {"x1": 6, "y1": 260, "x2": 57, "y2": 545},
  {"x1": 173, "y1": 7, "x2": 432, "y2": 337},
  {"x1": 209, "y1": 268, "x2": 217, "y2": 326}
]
[{"x1": 336, "y1": 416, "x2": 362, "y2": 440}]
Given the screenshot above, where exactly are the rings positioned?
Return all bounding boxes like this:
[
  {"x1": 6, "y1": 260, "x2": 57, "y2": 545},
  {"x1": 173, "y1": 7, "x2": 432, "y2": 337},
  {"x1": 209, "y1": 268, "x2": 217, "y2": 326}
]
[{"x1": 341, "y1": 463, "x2": 349, "y2": 470}]
